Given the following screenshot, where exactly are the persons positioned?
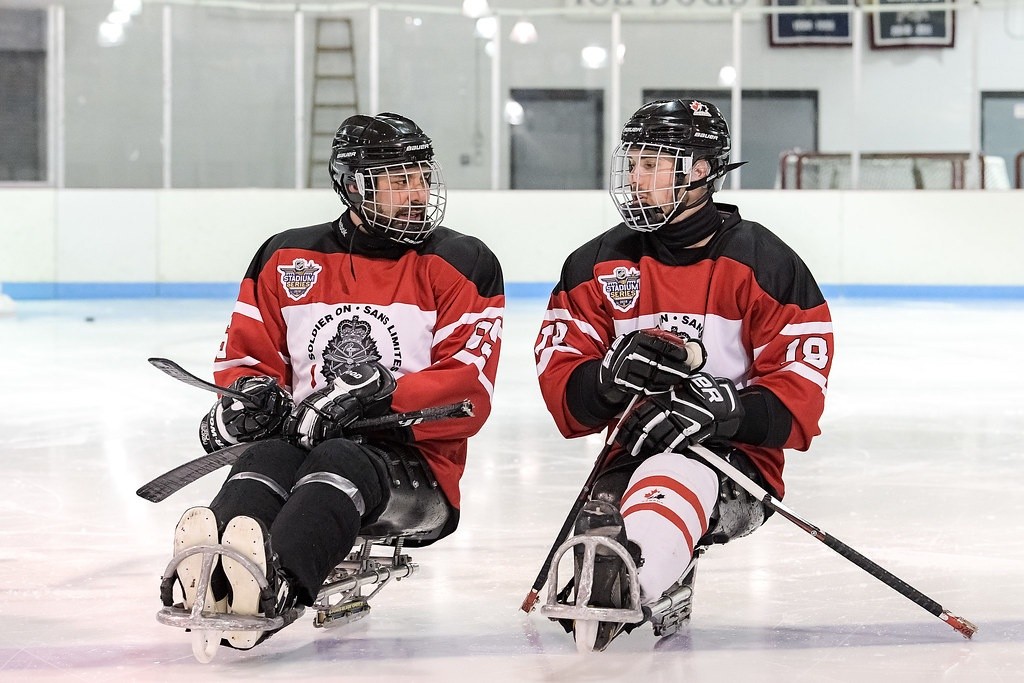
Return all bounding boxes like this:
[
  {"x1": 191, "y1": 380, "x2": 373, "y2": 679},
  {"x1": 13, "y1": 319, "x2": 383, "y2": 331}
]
[
  {"x1": 171, "y1": 110, "x2": 507, "y2": 651},
  {"x1": 532, "y1": 96, "x2": 835, "y2": 652}
]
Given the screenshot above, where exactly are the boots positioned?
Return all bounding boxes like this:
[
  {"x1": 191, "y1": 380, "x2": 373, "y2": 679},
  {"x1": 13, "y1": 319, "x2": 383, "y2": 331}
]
[
  {"x1": 172, "y1": 506, "x2": 302, "y2": 650},
  {"x1": 572, "y1": 499, "x2": 645, "y2": 653}
]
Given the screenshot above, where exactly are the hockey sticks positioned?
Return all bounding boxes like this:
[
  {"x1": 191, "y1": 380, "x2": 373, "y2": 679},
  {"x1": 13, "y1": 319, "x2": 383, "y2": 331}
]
[
  {"x1": 517, "y1": 393, "x2": 641, "y2": 615},
  {"x1": 688, "y1": 441, "x2": 979, "y2": 638},
  {"x1": 135, "y1": 399, "x2": 473, "y2": 504},
  {"x1": 149, "y1": 355, "x2": 290, "y2": 414}
]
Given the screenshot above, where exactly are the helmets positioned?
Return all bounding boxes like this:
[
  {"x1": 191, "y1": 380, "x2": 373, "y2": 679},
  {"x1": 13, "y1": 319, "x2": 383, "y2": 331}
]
[
  {"x1": 611, "y1": 99, "x2": 733, "y2": 232},
  {"x1": 327, "y1": 112, "x2": 445, "y2": 246}
]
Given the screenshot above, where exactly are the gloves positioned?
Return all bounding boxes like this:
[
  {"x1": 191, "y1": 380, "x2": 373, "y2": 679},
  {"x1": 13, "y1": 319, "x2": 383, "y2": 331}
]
[
  {"x1": 199, "y1": 376, "x2": 295, "y2": 456},
  {"x1": 598, "y1": 327, "x2": 705, "y2": 404},
  {"x1": 284, "y1": 361, "x2": 397, "y2": 451},
  {"x1": 615, "y1": 375, "x2": 740, "y2": 462}
]
